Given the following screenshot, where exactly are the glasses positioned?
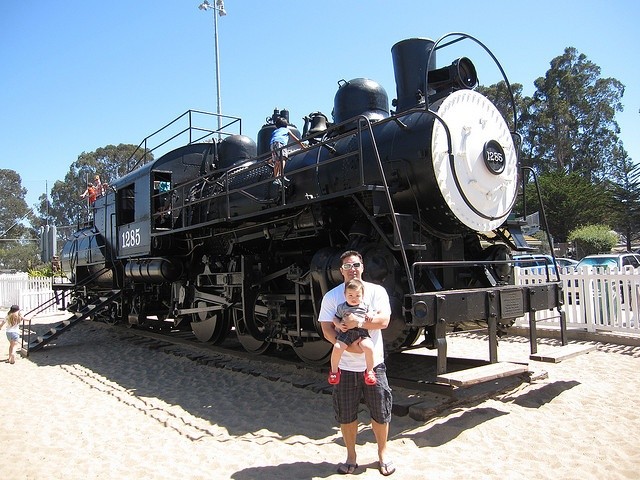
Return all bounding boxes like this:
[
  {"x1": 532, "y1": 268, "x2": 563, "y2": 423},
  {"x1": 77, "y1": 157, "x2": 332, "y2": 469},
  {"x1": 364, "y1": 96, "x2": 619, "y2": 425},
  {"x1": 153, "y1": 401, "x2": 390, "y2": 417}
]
[{"x1": 341, "y1": 262, "x2": 363, "y2": 270}]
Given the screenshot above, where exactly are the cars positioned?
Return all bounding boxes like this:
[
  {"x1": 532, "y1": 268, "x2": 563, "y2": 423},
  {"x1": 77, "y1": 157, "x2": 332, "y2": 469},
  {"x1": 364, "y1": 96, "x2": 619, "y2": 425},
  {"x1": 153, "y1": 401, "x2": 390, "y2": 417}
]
[{"x1": 554, "y1": 257, "x2": 580, "y2": 273}]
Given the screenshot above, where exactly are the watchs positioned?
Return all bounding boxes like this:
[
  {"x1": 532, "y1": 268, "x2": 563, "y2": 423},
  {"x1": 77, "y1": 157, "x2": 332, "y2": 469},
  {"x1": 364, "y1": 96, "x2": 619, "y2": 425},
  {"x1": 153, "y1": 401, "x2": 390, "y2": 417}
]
[{"x1": 358, "y1": 317, "x2": 362, "y2": 328}]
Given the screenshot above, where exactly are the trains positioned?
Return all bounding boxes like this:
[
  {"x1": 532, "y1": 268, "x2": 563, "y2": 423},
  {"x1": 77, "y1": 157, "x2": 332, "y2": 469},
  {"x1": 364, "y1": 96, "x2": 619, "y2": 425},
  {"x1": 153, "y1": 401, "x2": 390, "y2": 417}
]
[{"x1": 22, "y1": 32, "x2": 568, "y2": 376}]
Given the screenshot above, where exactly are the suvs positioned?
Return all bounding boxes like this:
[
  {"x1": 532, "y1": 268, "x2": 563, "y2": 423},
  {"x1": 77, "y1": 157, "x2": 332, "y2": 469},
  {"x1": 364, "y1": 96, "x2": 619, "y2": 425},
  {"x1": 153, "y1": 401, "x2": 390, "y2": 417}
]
[
  {"x1": 569, "y1": 253, "x2": 640, "y2": 305},
  {"x1": 513, "y1": 253, "x2": 561, "y2": 284}
]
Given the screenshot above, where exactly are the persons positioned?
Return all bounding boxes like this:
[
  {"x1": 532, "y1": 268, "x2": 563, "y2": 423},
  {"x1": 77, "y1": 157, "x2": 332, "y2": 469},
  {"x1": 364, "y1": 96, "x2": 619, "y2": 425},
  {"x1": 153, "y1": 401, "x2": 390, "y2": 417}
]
[
  {"x1": 80, "y1": 183, "x2": 97, "y2": 205},
  {"x1": 317, "y1": 250, "x2": 396, "y2": 475},
  {"x1": 328, "y1": 278, "x2": 378, "y2": 385},
  {"x1": 0, "y1": 305, "x2": 30, "y2": 364},
  {"x1": 269, "y1": 117, "x2": 307, "y2": 186},
  {"x1": 93, "y1": 175, "x2": 109, "y2": 194}
]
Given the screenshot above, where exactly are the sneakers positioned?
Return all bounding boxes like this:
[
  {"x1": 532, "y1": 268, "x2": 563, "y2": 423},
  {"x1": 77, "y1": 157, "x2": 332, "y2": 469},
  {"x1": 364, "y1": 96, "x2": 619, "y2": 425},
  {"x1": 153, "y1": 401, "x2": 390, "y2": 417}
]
[
  {"x1": 280, "y1": 174, "x2": 291, "y2": 182},
  {"x1": 364, "y1": 369, "x2": 377, "y2": 385},
  {"x1": 273, "y1": 179, "x2": 282, "y2": 188},
  {"x1": 328, "y1": 367, "x2": 342, "y2": 384}
]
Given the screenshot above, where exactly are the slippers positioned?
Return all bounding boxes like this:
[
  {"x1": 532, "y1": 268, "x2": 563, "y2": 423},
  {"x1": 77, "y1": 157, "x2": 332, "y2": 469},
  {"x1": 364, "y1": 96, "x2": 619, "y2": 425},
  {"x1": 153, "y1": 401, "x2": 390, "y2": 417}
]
[
  {"x1": 337, "y1": 463, "x2": 357, "y2": 474},
  {"x1": 378, "y1": 462, "x2": 396, "y2": 476}
]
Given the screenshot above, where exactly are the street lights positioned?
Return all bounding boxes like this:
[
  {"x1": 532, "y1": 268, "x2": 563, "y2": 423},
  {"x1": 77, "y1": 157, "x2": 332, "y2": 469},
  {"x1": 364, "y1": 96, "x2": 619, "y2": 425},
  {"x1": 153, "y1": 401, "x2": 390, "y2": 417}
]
[{"x1": 198, "y1": 0, "x2": 227, "y2": 142}]
